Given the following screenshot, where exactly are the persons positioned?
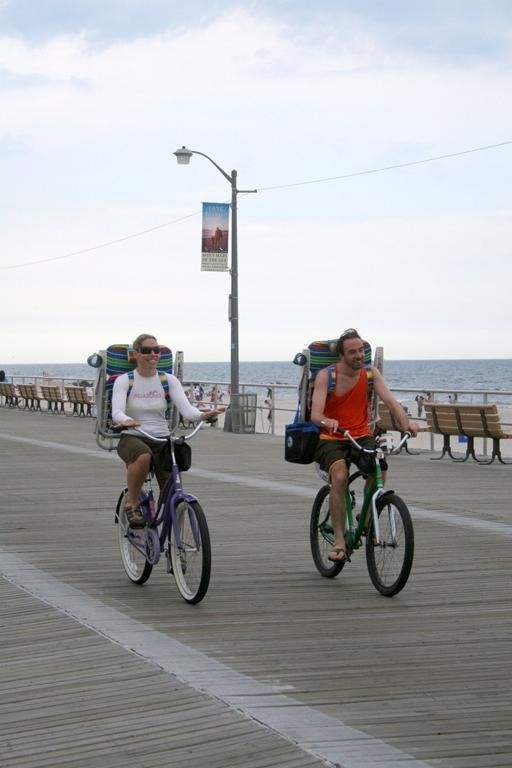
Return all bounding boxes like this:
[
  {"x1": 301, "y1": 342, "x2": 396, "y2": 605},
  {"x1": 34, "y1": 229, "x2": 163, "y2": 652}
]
[
  {"x1": 185, "y1": 383, "x2": 224, "y2": 409},
  {"x1": 306, "y1": 327, "x2": 419, "y2": 562},
  {"x1": 215, "y1": 226, "x2": 222, "y2": 246},
  {"x1": 210, "y1": 236, "x2": 214, "y2": 245},
  {"x1": 414, "y1": 392, "x2": 431, "y2": 417},
  {"x1": 264, "y1": 383, "x2": 273, "y2": 421},
  {"x1": 448, "y1": 393, "x2": 457, "y2": 404},
  {"x1": 111, "y1": 334, "x2": 228, "y2": 577},
  {"x1": 0, "y1": 370, "x2": 14, "y2": 408}
]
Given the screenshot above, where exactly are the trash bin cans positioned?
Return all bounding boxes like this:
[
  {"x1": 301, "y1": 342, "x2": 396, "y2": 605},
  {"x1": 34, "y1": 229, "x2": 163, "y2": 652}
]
[{"x1": 230, "y1": 394, "x2": 257, "y2": 434}]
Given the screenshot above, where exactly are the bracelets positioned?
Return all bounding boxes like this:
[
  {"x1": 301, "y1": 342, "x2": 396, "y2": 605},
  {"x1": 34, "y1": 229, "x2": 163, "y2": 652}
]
[{"x1": 320, "y1": 418, "x2": 327, "y2": 428}]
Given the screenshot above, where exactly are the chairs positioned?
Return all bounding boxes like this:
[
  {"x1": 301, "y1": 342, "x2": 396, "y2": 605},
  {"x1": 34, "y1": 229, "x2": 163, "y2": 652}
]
[
  {"x1": 93, "y1": 343, "x2": 184, "y2": 450},
  {"x1": 297, "y1": 339, "x2": 385, "y2": 434}
]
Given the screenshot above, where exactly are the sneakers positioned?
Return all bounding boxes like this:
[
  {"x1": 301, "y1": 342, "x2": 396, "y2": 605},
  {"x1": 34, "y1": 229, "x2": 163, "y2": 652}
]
[{"x1": 123, "y1": 504, "x2": 148, "y2": 530}]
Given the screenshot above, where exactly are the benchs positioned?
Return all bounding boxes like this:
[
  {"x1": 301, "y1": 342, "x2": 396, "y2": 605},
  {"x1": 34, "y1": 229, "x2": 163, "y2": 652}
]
[
  {"x1": 178, "y1": 409, "x2": 211, "y2": 429},
  {"x1": 375, "y1": 399, "x2": 428, "y2": 455},
  {"x1": 423, "y1": 402, "x2": 512, "y2": 465},
  {"x1": 0, "y1": 382, "x2": 97, "y2": 418}
]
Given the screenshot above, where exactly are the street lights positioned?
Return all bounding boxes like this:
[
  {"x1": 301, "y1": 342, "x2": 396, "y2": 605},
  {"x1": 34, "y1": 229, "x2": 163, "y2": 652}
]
[{"x1": 171, "y1": 144, "x2": 247, "y2": 432}]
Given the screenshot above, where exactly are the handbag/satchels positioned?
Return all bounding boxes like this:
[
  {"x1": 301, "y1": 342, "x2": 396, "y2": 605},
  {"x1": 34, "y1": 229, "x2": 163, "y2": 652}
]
[
  {"x1": 284, "y1": 422, "x2": 320, "y2": 465},
  {"x1": 150, "y1": 434, "x2": 192, "y2": 472}
]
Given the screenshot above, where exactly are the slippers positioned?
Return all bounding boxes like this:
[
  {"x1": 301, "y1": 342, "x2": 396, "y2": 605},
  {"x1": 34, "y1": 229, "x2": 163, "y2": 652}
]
[{"x1": 326, "y1": 547, "x2": 348, "y2": 564}]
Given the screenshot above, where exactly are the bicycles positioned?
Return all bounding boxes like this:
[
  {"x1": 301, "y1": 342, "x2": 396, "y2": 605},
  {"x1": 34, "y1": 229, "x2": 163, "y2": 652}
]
[
  {"x1": 111, "y1": 408, "x2": 219, "y2": 606},
  {"x1": 306, "y1": 418, "x2": 418, "y2": 599}
]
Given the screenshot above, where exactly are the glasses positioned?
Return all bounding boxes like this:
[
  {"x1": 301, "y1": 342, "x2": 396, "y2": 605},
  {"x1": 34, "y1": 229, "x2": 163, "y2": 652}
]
[{"x1": 132, "y1": 346, "x2": 160, "y2": 355}]
